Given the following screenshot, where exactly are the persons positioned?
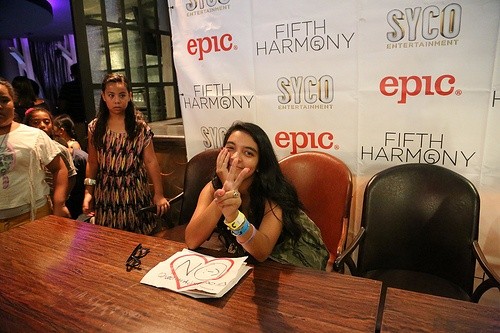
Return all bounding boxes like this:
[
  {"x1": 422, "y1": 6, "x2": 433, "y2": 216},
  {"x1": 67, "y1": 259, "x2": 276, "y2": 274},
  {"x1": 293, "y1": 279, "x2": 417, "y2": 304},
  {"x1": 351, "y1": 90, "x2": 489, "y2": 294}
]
[
  {"x1": 0, "y1": 62, "x2": 87, "y2": 235},
  {"x1": 82, "y1": 72, "x2": 171, "y2": 236},
  {"x1": 184, "y1": 121, "x2": 330, "y2": 275}
]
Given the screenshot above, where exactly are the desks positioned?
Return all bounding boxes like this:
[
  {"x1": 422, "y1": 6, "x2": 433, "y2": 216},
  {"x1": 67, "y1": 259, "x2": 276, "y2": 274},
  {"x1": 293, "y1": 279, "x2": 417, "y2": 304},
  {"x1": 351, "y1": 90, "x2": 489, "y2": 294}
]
[
  {"x1": 0, "y1": 214, "x2": 383, "y2": 333},
  {"x1": 379, "y1": 287, "x2": 500, "y2": 333}
]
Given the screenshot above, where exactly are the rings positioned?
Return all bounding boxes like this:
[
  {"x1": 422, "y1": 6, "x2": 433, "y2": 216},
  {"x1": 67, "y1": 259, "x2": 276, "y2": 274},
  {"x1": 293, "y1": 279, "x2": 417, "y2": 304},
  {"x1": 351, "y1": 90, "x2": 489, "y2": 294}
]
[{"x1": 233, "y1": 191, "x2": 239, "y2": 198}]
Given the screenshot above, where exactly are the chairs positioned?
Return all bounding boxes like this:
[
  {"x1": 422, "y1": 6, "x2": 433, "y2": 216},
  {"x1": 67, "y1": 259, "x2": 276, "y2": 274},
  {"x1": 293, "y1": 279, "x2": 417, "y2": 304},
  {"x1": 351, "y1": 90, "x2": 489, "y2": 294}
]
[
  {"x1": 136, "y1": 148, "x2": 227, "y2": 254},
  {"x1": 276, "y1": 151, "x2": 353, "y2": 275},
  {"x1": 333, "y1": 163, "x2": 500, "y2": 303}
]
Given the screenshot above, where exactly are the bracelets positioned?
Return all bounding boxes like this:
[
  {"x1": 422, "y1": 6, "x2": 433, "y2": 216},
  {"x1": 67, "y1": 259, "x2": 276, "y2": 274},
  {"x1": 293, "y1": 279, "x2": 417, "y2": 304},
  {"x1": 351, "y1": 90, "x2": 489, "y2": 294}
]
[
  {"x1": 224, "y1": 210, "x2": 257, "y2": 247},
  {"x1": 84, "y1": 178, "x2": 96, "y2": 186}
]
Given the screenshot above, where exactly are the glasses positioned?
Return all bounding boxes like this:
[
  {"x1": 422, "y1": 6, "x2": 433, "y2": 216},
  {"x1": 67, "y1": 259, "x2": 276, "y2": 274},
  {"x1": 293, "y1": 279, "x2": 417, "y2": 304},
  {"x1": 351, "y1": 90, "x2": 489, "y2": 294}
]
[{"x1": 126, "y1": 243, "x2": 150, "y2": 266}]
